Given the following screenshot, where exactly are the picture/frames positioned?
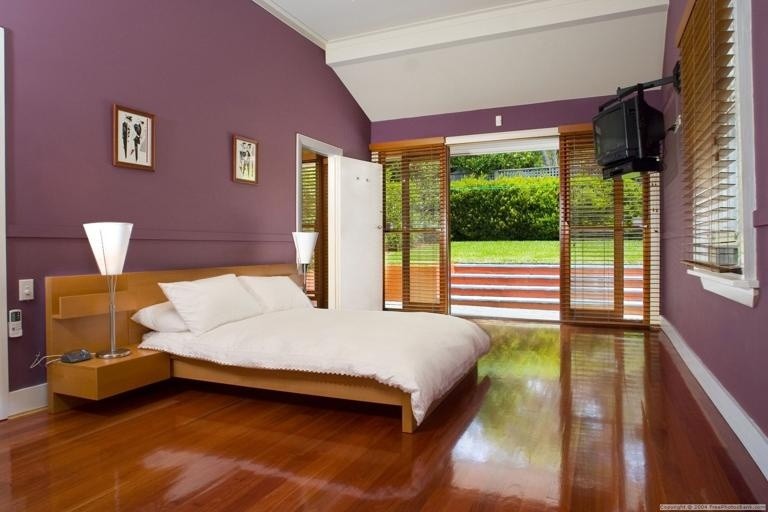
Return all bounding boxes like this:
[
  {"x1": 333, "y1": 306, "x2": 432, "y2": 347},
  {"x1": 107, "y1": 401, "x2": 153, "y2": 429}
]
[
  {"x1": 112, "y1": 103, "x2": 158, "y2": 171},
  {"x1": 232, "y1": 133, "x2": 261, "y2": 187}
]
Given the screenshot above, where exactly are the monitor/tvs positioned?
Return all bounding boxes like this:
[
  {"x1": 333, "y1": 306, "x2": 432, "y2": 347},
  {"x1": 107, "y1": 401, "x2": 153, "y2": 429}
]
[{"x1": 591, "y1": 99, "x2": 665, "y2": 167}]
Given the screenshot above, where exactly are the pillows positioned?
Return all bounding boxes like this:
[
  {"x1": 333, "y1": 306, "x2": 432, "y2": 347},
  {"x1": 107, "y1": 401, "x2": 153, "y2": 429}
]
[{"x1": 130, "y1": 273, "x2": 314, "y2": 337}]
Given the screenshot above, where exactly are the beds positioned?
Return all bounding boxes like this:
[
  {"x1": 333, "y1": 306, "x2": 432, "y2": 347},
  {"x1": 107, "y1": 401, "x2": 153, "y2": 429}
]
[{"x1": 139, "y1": 305, "x2": 492, "y2": 435}]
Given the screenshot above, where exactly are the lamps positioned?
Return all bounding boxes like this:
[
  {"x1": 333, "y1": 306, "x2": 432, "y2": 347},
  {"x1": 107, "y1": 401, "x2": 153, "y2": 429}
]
[
  {"x1": 82, "y1": 220, "x2": 137, "y2": 358},
  {"x1": 292, "y1": 232, "x2": 320, "y2": 292}
]
